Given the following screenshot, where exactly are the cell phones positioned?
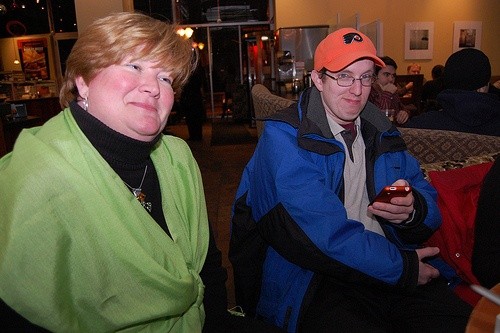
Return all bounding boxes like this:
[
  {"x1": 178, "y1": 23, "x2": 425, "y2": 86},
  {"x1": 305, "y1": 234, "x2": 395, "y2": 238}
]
[{"x1": 368, "y1": 186, "x2": 412, "y2": 207}]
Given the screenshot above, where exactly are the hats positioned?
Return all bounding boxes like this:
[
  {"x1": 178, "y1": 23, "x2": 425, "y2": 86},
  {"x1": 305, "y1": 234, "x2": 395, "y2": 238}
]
[{"x1": 313, "y1": 27, "x2": 385, "y2": 73}]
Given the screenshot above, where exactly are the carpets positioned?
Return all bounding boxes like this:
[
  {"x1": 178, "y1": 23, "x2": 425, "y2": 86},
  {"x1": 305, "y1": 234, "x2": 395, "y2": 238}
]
[{"x1": 208, "y1": 121, "x2": 258, "y2": 146}]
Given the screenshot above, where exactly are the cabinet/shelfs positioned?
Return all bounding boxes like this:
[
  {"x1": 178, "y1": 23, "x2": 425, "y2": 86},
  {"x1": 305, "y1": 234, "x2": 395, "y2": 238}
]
[{"x1": 6, "y1": 96, "x2": 63, "y2": 126}]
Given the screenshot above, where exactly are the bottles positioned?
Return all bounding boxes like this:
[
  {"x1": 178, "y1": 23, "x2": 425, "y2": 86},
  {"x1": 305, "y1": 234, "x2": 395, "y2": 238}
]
[{"x1": 10, "y1": 104, "x2": 18, "y2": 117}]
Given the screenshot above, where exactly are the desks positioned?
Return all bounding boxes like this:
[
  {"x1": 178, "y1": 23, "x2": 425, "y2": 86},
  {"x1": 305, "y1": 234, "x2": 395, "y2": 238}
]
[
  {"x1": 2, "y1": 114, "x2": 41, "y2": 154},
  {"x1": 394, "y1": 74, "x2": 424, "y2": 87}
]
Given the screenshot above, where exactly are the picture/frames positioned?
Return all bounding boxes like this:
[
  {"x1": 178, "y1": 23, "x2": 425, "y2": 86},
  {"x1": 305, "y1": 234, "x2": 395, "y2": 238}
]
[
  {"x1": 404, "y1": 22, "x2": 433, "y2": 60},
  {"x1": 452, "y1": 20, "x2": 483, "y2": 54}
]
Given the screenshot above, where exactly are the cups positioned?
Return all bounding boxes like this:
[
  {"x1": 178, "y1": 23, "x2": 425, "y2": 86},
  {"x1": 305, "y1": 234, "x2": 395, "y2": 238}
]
[{"x1": 381, "y1": 109, "x2": 397, "y2": 126}]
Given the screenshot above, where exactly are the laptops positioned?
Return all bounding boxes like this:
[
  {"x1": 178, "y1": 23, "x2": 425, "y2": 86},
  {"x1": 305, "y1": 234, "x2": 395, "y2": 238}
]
[{"x1": 15, "y1": 104, "x2": 28, "y2": 118}]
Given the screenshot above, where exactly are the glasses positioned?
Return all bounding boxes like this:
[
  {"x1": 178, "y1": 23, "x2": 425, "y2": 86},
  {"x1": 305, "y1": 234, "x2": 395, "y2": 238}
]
[{"x1": 322, "y1": 73, "x2": 377, "y2": 87}]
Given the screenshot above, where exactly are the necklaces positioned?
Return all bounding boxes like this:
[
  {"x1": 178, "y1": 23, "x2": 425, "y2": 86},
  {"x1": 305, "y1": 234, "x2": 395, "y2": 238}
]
[{"x1": 123, "y1": 165, "x2": 152, "y2": 212}]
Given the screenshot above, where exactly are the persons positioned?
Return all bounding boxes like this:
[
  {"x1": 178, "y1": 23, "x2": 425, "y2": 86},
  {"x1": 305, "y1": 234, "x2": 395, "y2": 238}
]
[
  {"x1": 179, "y1": 57, "x2": 209, "y2": 142},
  {"x1": 407, "y1": 49, "x2": 500, "y2": 134},
  {"x1": 368, "y1": 56, "x2": 410, "y2": 124},
  {"x1": 424, "y1": 65, "x2": 444, "y2": 86},
  {"x1": 407, "y1": 62, "x2": 421, "y2": 75},
  {"x1": 227, "y1": 28, "x2": 475, "y2": 333},
  {"x1": 0, "y1": 12, "x2": 221, "y2": 333}
]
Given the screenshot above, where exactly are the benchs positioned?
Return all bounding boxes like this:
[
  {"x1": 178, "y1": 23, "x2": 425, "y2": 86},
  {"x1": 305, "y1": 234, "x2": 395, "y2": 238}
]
[{"x1": 252, "y1": 83, "x2": 500, "y2": 307}]
